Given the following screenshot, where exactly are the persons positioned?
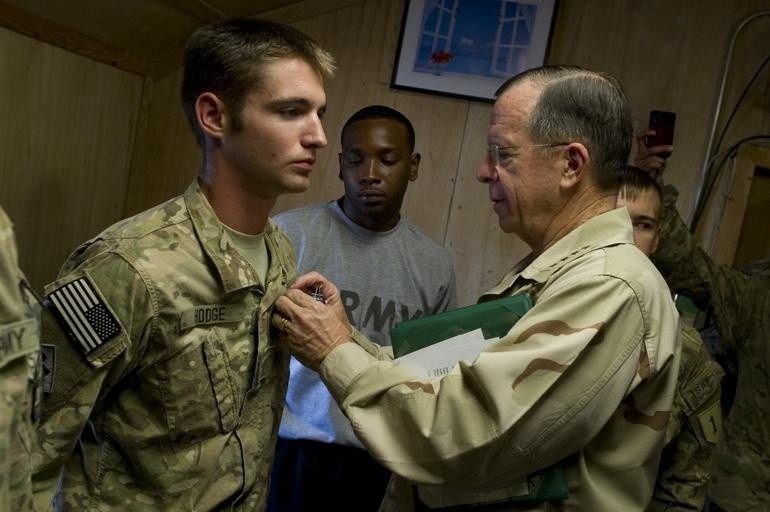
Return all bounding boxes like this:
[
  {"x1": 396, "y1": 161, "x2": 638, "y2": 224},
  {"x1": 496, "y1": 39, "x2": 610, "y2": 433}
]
[
  {"x1": 33, "y1": 17, "x2": 338, "y2": 512},
  {"x1": 635, "y1": 110, "x2": 770, "y2": 511},
  {"x1": 0, "y1": 206, "x2": 44, "y2": 512},
  {"x1": 271, "y1": 64, "x2": 682, "y2": 511},
  {"x1": 266, "y1": 106, "x2": 458, "y2": 511},
  {"x1": 616, "y1": 167, "x2": 725, "y2": 512}
]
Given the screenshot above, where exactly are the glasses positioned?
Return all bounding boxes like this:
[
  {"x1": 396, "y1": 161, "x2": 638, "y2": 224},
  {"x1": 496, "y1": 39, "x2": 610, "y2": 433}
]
[{"x1": 486, "y1": 142, "x2": 570, "y2": 160}]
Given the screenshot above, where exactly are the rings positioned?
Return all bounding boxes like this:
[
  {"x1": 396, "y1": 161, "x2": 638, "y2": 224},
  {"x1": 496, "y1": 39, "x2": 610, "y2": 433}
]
[{"x1": 279, "y1": 318, "x2": 288, "y2": 331}]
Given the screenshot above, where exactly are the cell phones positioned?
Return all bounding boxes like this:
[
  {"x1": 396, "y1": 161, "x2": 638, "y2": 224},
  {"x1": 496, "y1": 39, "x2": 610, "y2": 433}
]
[{"x1": 647, "y1": 110, "x2": 676, "y2": 158}]
